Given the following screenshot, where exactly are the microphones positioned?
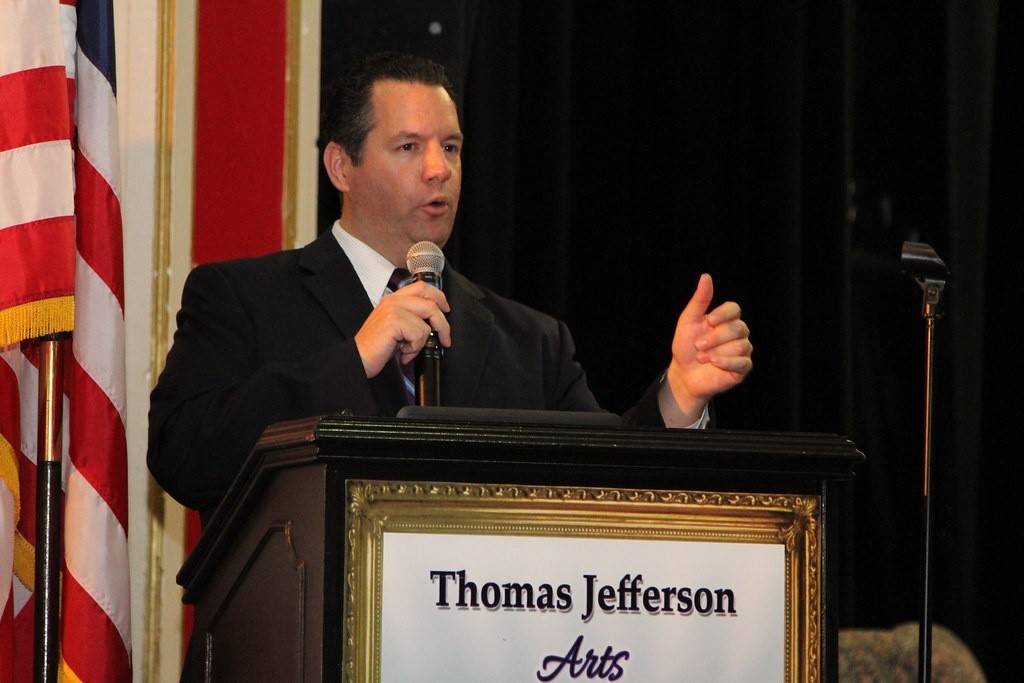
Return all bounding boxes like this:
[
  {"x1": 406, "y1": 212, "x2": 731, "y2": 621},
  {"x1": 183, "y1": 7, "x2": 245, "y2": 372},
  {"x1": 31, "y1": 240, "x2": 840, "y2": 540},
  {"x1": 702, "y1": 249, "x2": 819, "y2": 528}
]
[{"x1": 405, "y1": 241, "x2": 446, "y2": 408}]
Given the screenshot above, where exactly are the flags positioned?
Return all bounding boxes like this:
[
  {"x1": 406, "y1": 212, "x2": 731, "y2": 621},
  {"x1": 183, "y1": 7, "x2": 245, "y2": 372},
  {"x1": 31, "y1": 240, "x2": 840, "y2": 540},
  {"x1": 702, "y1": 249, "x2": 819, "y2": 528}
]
[{"x1": 0, "y1": 0, "x2": 133, "y2": 683}]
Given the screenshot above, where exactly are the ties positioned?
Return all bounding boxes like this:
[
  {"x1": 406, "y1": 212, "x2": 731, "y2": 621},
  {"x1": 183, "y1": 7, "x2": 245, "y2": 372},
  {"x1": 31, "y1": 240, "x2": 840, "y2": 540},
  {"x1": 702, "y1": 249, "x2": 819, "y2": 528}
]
[{"x1": 387, "y1": 268, "x2": 416, "y2": 408}]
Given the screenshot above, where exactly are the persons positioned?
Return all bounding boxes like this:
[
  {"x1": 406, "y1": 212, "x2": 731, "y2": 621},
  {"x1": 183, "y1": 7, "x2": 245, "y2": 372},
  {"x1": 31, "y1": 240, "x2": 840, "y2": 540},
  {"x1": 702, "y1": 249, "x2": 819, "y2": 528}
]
[{"x1": 147, "y1": 49, "x2": 756, "y2": 683}]
